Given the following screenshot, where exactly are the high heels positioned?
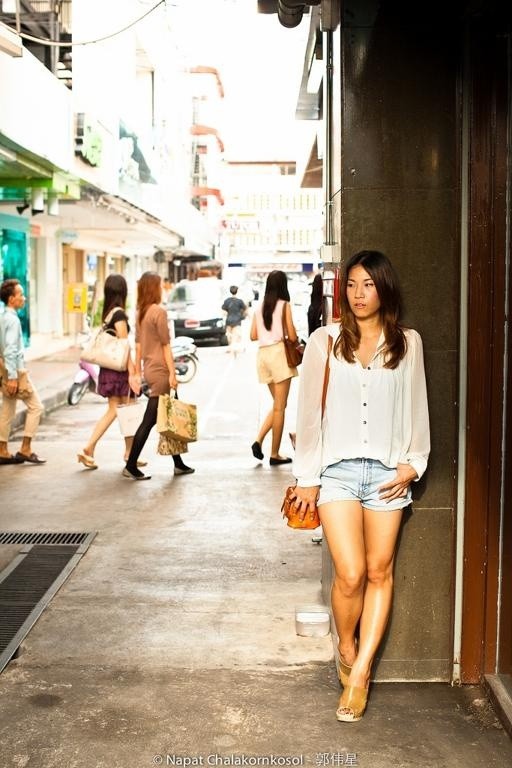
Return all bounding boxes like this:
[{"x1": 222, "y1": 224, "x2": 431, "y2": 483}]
[{"x1": 76, "y1": 454, "x2": 99, "y2": 470}]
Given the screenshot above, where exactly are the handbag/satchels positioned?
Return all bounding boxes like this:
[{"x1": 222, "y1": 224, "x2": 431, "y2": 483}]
[
  {"x1": 155, "y1": 391, "x2": 201, "y2": 444},
  {"x1": 0, "y1": 354, "x2": 32, "y2": 402},
  {"x1": 115, "y1": 401, "x2": 148, "y2": 438},
  {"x1": 156, "y1": 432, "x2": 189, "y2": 457},
  {"x1": 283, "y1": 337, "x2": 307, "y2": 369},
  {"x1": 280, "y1": 477, "x2": 322, "y2": 532},
  {"x1": 78, "y1": 307, "x2": 131, "y2": 374}
]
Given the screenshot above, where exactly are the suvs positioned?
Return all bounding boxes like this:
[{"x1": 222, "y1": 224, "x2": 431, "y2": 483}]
[{"x1": 162, "y1": 276, "x2": 230, "y2": 352}]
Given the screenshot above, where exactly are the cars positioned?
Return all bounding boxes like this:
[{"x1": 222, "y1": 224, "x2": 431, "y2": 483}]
[{"x1": 225, "y1": 270, "x2": 261, "y2": 312}]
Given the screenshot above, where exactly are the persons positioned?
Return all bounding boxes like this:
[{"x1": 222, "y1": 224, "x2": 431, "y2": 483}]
[
  {"x1": 308, "y1": 274, "x2": 324, "y2": 335},
  {"x1": 122, "y1": 270, "x2": 195, "y2": 481},
  {"x1": 76, "y1": 272, "x2": 146, "y2": 470},
  {"x1": 222, "y1": 286, "x2": 246, "y2": 353},
  {"x1": 251, "y1": 269, "x2": 298, "y2": 467},
  {"x1": 0, "y1": 279, "x2": 46, "y2": 465},
  {"x1": 287, "y1": 250, "x2": 432, "y2": 722}
]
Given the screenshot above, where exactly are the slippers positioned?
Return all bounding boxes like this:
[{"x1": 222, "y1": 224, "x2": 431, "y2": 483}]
[{"x1": 0, "y1": 454, "x2": 25, "y2": 464}]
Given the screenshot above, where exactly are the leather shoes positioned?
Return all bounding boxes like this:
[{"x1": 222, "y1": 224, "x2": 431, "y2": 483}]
[
  {"x1": 250, "y1": 441, "x2": 264, "y2": 460},
  {"x1": 15, "y1": 451, "x2": 48, "y2": 464},
  {"x1": 173, "y1": 465, "x2": 196, "y2": 476},
  {"x1": 122, "y1": 466, "x2": 152, "y2": 481},
  {"x1": 269, "y1": 456, "x2": 294, "y2": 466}
]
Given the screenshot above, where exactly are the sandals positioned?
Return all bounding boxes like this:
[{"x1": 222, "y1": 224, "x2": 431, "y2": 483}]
[{"x1": 335, "y1": 650, "x2": 370, "y2": 725}]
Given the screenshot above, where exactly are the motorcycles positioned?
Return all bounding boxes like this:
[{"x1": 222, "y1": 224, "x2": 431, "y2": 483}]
[{"x1": 62, "y1": 313, "x2": 203, "y2": 409}]
